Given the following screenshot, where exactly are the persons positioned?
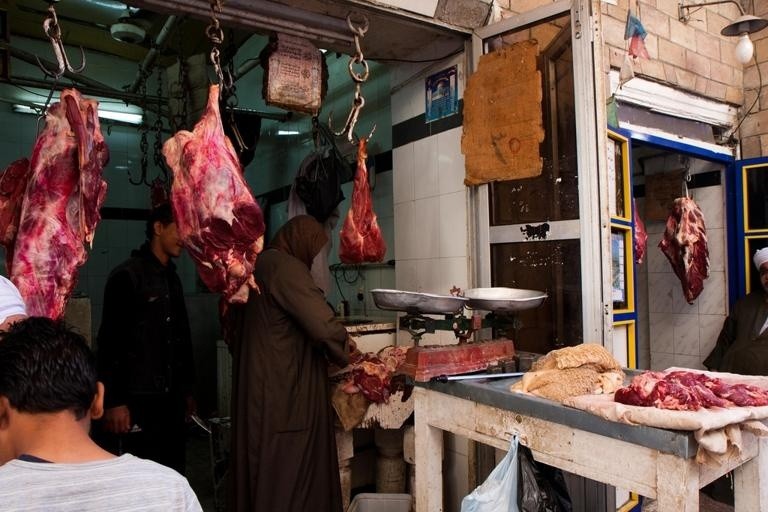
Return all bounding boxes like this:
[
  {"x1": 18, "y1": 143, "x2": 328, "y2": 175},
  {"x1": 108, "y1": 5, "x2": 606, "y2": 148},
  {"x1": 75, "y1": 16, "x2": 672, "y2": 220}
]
[
  {"x1": 90, "y1": 203, "x2": 204, "y2": 470},
  {"x1": 703, "y1": 248, "x2": 768, "y2": 377},
  {"x1": 225, "y1": 215, "x2": 360, "y2": 510},
  {"x1": 0, "y1": 315, "x2": 207, "y2": 511}
]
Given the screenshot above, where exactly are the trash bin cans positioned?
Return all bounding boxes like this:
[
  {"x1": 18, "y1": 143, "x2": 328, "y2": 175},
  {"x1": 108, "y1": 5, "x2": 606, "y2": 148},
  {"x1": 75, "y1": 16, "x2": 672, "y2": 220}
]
[{"x1": 346, "y1": 493, "x2": 412, "y2": 512}]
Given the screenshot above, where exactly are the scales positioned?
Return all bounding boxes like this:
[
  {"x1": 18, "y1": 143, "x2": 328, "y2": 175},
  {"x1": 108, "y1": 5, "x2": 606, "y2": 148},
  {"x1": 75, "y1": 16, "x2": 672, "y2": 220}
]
[{"x1": 368, "y1": 287, "x2": 550, "y2": 383}]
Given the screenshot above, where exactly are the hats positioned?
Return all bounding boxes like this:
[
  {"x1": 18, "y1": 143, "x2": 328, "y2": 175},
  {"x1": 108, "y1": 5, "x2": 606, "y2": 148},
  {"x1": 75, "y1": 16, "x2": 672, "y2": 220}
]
[{"x1": 753, "y1": 246, "x2": 768, "y2": 272}]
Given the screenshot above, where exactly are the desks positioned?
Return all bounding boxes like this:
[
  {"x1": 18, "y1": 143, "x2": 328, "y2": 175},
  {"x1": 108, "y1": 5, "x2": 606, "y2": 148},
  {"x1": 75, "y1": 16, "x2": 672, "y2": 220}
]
[{"x1": 403, "y1": 347, "x2": 767, "y2": 511}]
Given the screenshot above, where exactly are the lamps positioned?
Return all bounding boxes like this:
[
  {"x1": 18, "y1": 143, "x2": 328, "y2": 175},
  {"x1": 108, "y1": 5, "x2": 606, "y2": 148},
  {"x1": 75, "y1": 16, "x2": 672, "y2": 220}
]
[{"x1": 678, "y1": 0, "x2": 768, "y2": 63}]
